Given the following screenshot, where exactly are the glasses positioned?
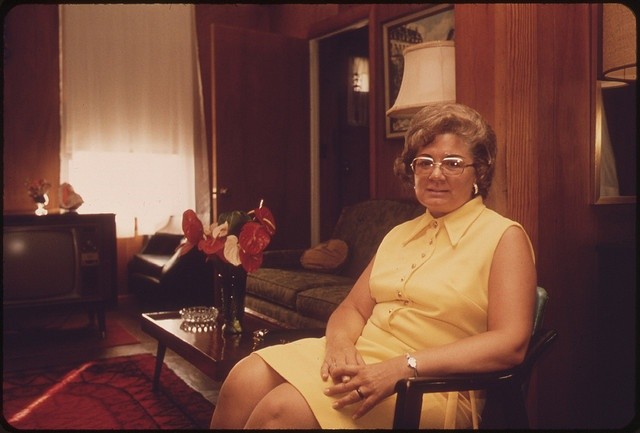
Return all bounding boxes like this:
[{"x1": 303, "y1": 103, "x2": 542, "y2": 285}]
[{"x1": 409, "y1": 157, "x2": 477, "y2": 176}]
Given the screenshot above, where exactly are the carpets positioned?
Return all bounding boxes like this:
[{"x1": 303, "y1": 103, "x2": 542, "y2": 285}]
[
  {"x1": 2, "y1": 302, "x2": 139, "y2": 372},
  {"x1": 0, "y1": 353, "x2": 215, "y2": 431}
]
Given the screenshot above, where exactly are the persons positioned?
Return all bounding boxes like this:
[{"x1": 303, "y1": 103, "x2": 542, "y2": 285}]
[{"x1": 209, "y1": 102, "x2": 539, "y2": 431}]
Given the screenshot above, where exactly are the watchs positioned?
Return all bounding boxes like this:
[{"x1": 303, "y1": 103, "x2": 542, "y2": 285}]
[{"x1": 402, "y1": 349, "x2": 420, "y2": 379}]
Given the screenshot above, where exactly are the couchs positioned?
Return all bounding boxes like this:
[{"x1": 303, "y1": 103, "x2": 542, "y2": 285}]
[
  {"x1": 243, "y1": 200, "x2": 427, "y2": 344},
  {"x1": 127, "y1": 233, "x2": 213, "y2": 310}
]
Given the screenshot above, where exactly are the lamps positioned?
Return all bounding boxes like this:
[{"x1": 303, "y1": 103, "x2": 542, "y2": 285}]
[{"x1": 386, "y1": 41, "x2": 457, "y2": 119}]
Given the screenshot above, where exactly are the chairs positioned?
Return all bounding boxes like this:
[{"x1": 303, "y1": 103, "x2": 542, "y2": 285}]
[{"x1": 249, "y1": 287, "x2": 559, "y2": 429}]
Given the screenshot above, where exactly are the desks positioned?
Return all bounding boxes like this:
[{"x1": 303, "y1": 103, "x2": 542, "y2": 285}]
[
  {"x1": 140, "y1": 307, "x2": 303, "y2": 385},
  {"x1": 1, "y1": 301, "x2": 115, "y2": 340}
]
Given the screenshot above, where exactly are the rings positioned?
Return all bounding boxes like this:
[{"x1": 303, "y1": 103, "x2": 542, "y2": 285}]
[{"x1": 354, "y1": 386, "x2": 366, "y2": 400}]
